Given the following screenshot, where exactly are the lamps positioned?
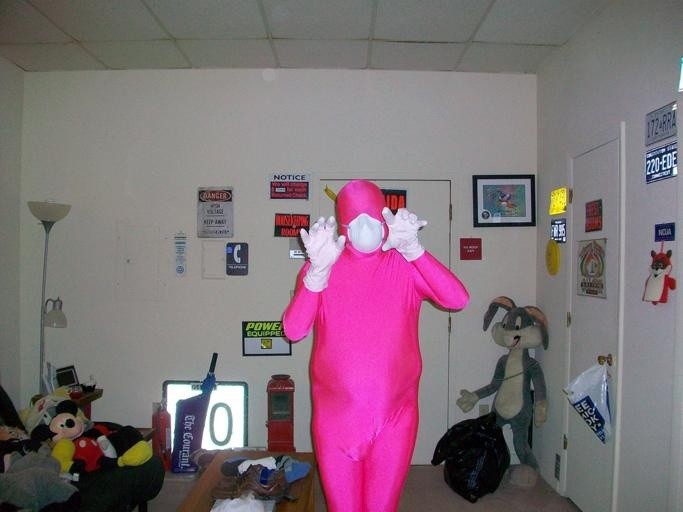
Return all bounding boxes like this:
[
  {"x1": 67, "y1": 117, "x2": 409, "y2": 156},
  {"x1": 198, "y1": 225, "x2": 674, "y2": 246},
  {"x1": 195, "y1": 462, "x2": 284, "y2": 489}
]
[{"x1": 27, "y1": 201, "x2": 71, "y2": 394}]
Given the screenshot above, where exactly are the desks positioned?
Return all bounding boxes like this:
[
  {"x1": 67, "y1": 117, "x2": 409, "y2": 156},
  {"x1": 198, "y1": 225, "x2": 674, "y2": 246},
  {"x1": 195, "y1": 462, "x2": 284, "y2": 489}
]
[{"x1": 32, "y1": 384, "x2": 103, "y2": 421}]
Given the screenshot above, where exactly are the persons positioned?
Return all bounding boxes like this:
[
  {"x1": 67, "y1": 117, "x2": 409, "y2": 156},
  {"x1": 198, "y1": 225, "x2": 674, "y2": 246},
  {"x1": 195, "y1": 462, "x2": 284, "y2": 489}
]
[{"x1": 282, "y1": 177, "x2": 469, "y2": 512}]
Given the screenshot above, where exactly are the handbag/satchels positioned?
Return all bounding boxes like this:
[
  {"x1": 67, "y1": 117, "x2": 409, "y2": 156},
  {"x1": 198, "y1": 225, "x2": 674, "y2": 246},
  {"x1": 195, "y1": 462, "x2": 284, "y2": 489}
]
[{"x1": 431, "y1": 410, "x2": 511, "y2": 502}]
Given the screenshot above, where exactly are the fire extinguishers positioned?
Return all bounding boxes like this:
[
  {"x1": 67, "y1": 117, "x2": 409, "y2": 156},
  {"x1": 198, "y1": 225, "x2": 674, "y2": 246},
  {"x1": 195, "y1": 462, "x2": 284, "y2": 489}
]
[{"x1": 152, "y1": 397, "x2": 173, "y2": 473}]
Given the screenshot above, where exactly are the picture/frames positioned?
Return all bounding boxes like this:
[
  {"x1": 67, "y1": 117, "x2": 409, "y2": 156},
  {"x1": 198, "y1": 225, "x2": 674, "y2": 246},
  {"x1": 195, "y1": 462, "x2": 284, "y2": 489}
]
[
  {"x1": 473, "y1": 174, "x2": 536, "y2": 227},
  {"x1": 162, "y1": 380, "x2": 248, "y2": 450},
  {"x1": 56, "y1": 365, "x2": 79, "y2": 390}
]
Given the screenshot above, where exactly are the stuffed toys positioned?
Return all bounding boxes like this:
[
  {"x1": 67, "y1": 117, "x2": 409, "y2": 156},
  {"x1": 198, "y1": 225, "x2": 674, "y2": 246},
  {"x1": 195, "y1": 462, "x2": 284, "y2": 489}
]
[
  {"x1": 642, "y1": 249, "x2": 676, "y2": 306},
  {"x1": 455, "y1": 296, "x2": 549, "y2": 488},
  {"x1": 0, "y1": 394, "x2": 152, "y2": 512}
]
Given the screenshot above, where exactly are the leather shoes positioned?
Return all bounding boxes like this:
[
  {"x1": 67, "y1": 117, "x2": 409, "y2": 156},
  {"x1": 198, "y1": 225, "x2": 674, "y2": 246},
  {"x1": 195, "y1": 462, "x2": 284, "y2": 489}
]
[
  {"x1": 211, "y1": 473, "x2": 286, "y2": 501},
  {"x1": 219, "y1": 464, "x2": 287, "y2": 488}
]
